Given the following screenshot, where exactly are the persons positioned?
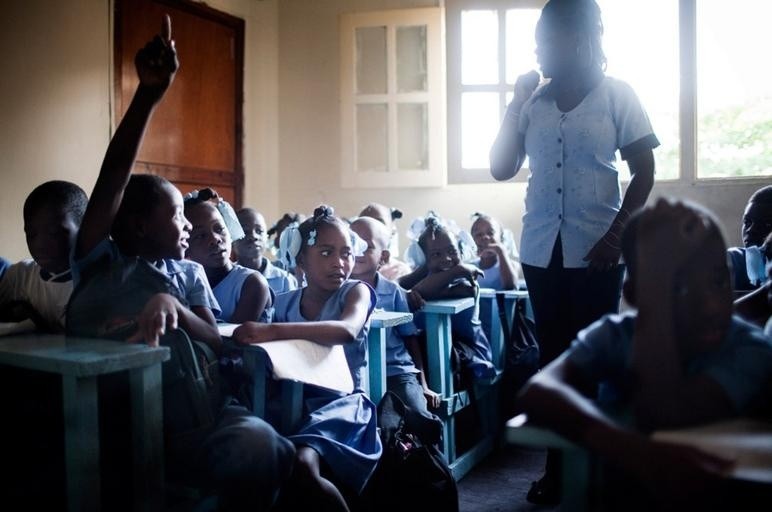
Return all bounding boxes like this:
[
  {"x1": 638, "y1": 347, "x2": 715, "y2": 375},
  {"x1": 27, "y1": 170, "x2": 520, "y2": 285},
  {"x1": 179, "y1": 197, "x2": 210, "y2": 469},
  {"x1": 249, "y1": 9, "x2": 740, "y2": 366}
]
[
  {"x1": 62, "y1": 12, "x2": 296, "y2": 512},
  {"x1": 0, "y1": 178, "x2": 771, "y2": 511},
  {"x1": 486, "y1": 1, "x2": 660, "y2": 370}
]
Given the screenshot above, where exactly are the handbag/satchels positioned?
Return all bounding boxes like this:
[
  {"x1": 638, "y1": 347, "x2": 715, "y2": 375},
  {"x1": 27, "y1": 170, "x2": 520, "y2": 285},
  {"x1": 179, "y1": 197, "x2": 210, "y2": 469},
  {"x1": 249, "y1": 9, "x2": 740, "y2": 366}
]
[
  {"x1": 499, "y1": 305, "x2": 540, "y2": 411},
  {"x1": 369, "y1": 392, "x2": 458, "y2": 512},
  {"x1": 106, "y1": 317, "x2": 220, "y2": 439}
]
